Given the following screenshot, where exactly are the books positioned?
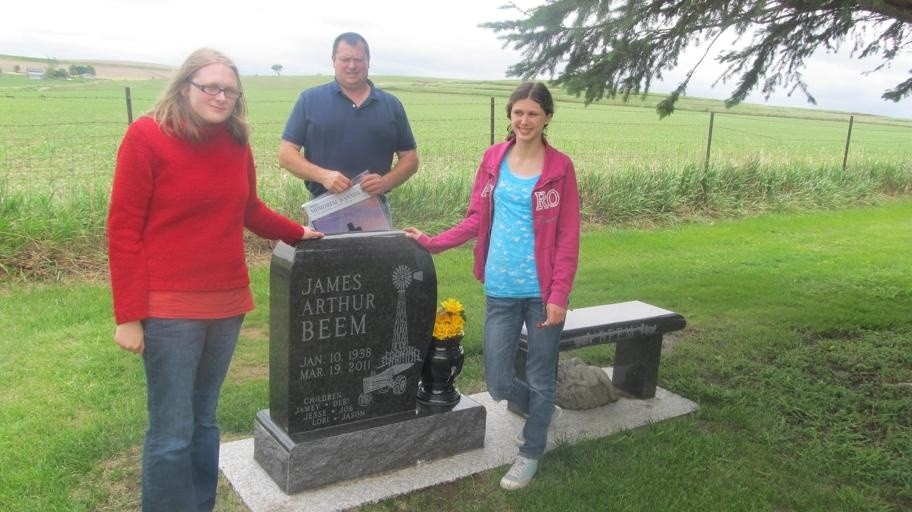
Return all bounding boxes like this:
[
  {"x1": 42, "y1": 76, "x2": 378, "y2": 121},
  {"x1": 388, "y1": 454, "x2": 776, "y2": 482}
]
[{"x1": 306, "y1": 183, "x2": 390, "y2": 235}]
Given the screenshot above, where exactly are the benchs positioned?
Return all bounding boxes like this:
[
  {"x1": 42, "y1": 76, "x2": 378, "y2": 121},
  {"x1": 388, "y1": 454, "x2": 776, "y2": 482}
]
[{"x1": 506, "y1": 300, "x2": 688, "y2": 420}]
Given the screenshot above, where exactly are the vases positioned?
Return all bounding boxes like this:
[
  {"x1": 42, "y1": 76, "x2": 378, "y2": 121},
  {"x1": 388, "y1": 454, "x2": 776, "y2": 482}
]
[{"x1": 417, "y1": 332, "x2": 466, "y2": 407}]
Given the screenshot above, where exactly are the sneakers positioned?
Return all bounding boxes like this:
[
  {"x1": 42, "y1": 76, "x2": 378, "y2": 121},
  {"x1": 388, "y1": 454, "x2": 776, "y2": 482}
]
[
  {"x1": 514, "y1": 403, "x2": 563, "y2": 447},
  {"x1": 499, "y1": 453, "x2": 538, "y2": 490}
]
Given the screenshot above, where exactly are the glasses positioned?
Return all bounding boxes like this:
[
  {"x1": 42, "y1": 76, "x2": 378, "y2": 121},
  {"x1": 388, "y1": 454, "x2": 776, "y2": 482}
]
[{"x1": 187, "y1": 78, "x2": 243, "y2": 99}]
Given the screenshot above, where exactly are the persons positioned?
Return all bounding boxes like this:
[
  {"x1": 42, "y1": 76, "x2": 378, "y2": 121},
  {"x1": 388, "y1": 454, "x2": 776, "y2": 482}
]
[
  {"x1": 102, "y1": 48, "x2": 326, "y2": 512},
  {"x1": 274, "y1": 31, "x2": 420, "y2": 235},
  {"x1": 400, "y1": 80, "x2": 582, "y2": 489}
]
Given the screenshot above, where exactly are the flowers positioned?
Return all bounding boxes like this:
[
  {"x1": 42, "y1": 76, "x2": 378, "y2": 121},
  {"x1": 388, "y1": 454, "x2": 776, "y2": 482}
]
[{"x1": 432, "y1": 298, "x2": 468, "y2": 342}]
